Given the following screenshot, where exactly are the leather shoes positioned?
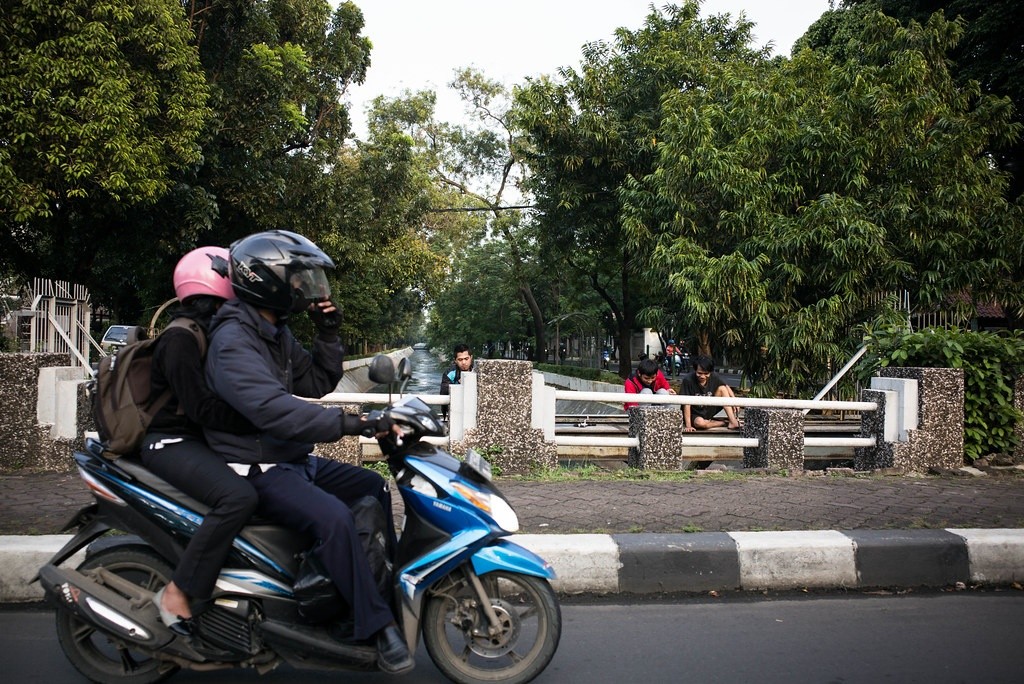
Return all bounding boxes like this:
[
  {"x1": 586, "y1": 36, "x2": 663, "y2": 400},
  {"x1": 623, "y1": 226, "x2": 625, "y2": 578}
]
[{"x1": 376, "y1": 625, "x2": 415, "y2": 677}]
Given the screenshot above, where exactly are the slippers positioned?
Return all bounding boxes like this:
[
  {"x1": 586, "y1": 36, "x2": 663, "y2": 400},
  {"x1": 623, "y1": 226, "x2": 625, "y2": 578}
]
[{"x1": 728, "y1": 426, "x2": 744, "y2": 431}]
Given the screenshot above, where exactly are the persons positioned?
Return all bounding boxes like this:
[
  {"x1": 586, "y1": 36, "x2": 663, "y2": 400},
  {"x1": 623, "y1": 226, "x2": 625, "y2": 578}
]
[
  {"x1": 623, "y1": 359, "x2": 681, "y2": 416},
  {"x1": 678, "y1": 354, "x2": 744, "y2": 433},
  {"x1": 603, "y1": 341, "x2": 608, "y2": 354},
  {"x1": 522, "y1": 340, "x2": 567, "y2": 362},
  {"x1": 666, "y1": 339, "x2": 691, "y2": 371},
  {"x1": 439, "y1": 343, "x2": 476, "y2": 424},
  {"x1": 141, "y1": 228, "x2": 416, "y2": 676}
]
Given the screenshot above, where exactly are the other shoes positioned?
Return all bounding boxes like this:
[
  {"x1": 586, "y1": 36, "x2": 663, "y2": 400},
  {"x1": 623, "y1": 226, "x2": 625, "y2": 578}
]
[{"x1": 151, "y1": 586, "x2": 199, "y2": 638}]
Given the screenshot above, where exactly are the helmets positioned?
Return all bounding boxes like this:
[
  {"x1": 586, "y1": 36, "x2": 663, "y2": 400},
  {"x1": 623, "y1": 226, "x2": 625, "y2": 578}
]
[
  {"x1": 227, "y1": 230, "x2": 335, "y2": 319},
  {"x1": 668, "y1": 340, "x2": 674, "y2": 344},
  {"x1": 173, "y1": 244, "x2": 235, "y2": 305}
]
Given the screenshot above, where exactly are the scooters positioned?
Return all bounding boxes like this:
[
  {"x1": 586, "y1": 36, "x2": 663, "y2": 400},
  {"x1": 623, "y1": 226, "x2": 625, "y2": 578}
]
[
  {"x1": 663, "y1": 351, "x2": 682, "y2": 376},
  {"x1": 26, "y1": 355, "x2": 565, "y2": 683},
  {"x1": 601, "y1": 351, "x2": 611, "y2": 370},
  {"x1": 682, "y1": 352, "x2": 692, "y2": 373}
]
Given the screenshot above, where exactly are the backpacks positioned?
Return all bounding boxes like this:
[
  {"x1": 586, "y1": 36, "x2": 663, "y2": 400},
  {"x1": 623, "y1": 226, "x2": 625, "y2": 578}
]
[{"x1": 92, "y1": 318, "x2": 207, "y2": 455}]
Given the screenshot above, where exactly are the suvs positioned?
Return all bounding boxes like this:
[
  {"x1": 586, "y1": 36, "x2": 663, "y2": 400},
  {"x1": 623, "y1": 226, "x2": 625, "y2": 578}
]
[{"x1": 99, "y1": 324, "x2": 141, "y2": 360}]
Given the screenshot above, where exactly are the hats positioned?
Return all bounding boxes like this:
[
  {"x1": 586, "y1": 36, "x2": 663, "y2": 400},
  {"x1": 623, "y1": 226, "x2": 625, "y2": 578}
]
[{"x1": 680, "y1": 341, "x2": 685, "y2": 343}]
